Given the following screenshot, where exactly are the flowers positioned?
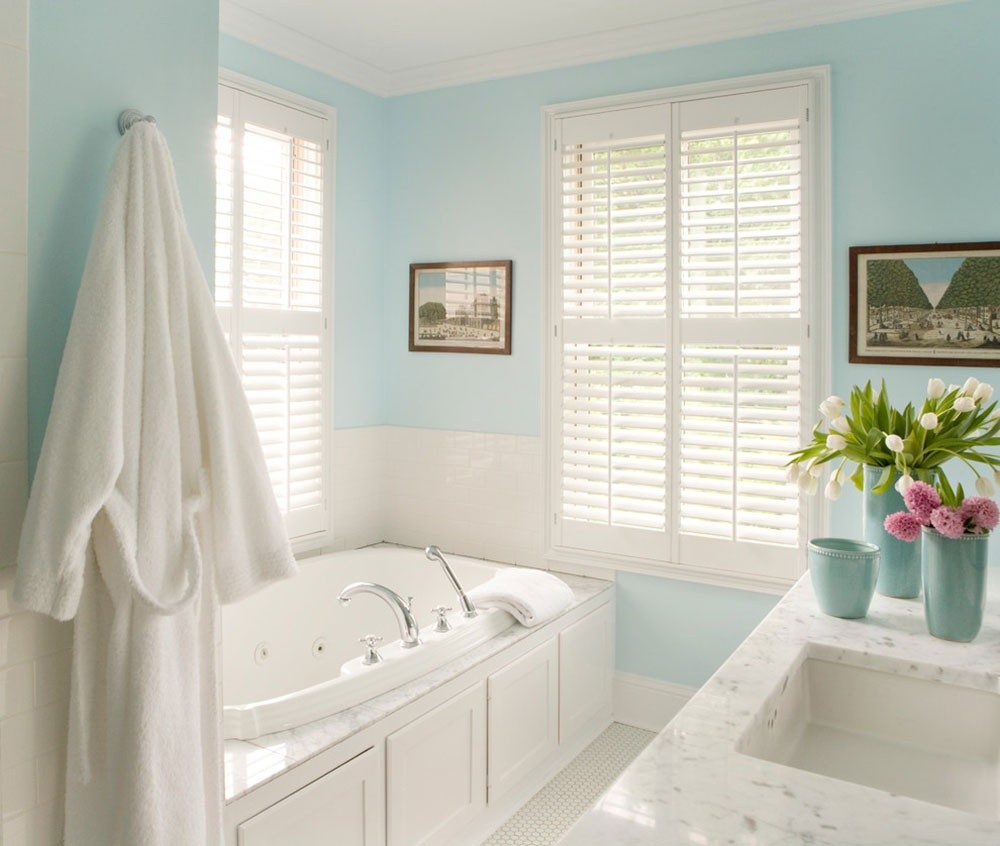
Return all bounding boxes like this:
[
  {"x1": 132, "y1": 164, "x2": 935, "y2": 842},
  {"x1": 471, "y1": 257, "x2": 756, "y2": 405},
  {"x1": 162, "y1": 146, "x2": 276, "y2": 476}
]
[
  {"x1": 883, "y1": 480, "x2": 1000, "y2": 542},
  {"x1": 783, "y1": 377, "x2": 1000, "y2": 499}
]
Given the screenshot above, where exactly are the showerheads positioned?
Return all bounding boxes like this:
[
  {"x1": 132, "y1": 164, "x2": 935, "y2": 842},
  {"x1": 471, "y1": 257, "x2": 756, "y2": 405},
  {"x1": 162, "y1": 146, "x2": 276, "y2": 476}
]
[{"x1": 425, "y1": 544, "x2": 442, "y2": 561}]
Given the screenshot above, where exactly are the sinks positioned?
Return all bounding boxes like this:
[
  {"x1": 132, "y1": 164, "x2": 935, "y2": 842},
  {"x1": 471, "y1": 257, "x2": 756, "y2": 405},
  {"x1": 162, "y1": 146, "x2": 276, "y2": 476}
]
[{"x1": 732, "y1": 640, "x2": 1000, "y2": 819}]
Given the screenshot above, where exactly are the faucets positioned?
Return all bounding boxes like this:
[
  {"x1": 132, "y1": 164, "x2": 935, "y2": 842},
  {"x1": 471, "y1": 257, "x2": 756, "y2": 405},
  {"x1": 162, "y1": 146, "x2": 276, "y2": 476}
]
[{"x1": 337, "y1": 582, "x2": 423, "y2": 649}]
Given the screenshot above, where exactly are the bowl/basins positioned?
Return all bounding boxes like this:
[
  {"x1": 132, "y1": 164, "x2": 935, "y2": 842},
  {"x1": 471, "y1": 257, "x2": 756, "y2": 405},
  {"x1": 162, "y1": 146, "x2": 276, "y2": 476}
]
[{"x1": 808, "y1": 536, "x2": 881, "y2": 620}]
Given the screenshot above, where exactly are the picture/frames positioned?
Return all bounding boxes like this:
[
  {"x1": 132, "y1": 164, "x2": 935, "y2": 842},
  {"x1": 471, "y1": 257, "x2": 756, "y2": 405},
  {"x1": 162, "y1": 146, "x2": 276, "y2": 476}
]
[
  {"x1": 849, "y1": 241, "x2": 1000, "y2": 368},
  {"x1": 409, "y1": 259, "x2": 512, "y2": 355}
]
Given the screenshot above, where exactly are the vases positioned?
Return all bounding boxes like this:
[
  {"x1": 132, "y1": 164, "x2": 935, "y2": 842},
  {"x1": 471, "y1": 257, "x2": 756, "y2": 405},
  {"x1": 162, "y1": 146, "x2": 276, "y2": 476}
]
[
  {"x1": 807, "y1": 537, "x2": 881, "y2": 621},
  {"x1": 921, "y1": 524, "x2": 990, "y2": 643},
  {"x1": 861, "y1": 462, "x2": 937, "y2": 600}
]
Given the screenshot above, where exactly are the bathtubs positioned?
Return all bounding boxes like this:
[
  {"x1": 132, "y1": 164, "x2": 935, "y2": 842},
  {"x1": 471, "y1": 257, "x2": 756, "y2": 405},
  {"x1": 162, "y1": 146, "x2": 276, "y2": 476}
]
[{"x1": 217, "y1": 545, "x2": 519, "y2": 741}]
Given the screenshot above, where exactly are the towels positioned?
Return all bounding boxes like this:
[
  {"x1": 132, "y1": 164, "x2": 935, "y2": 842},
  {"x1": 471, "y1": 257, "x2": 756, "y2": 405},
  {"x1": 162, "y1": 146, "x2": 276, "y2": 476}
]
[{"x1": 463, "y1": 567, "x2": 575, "y2": 628}]
